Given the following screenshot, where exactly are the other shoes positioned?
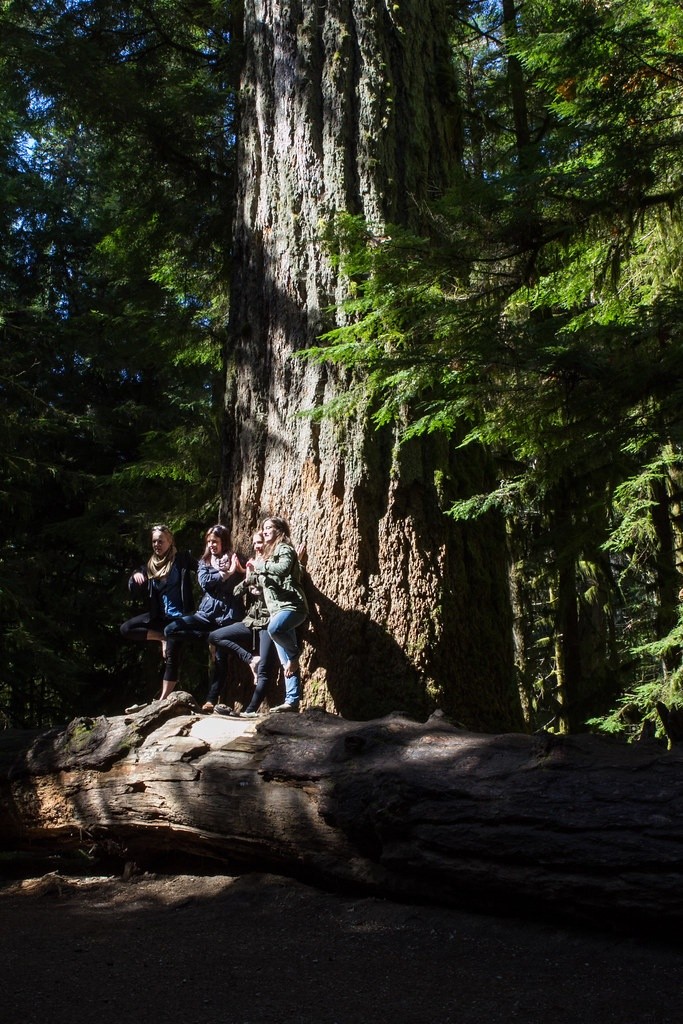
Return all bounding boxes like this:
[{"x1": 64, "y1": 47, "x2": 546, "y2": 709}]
[
  {"x1": 230, "y1": 700, "x2": 244, "y2": 717},
  {"x1": 201, "y1": 702, "x2": 214, "y2": 713},
  {"x1": 239, "y1": 712, "x2": 260, "y2": 718}
]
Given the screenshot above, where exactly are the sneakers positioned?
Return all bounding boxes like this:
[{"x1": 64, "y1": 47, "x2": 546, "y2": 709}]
[{"x1": 270, "y1": 705, "x2": 300, "y2": 713}]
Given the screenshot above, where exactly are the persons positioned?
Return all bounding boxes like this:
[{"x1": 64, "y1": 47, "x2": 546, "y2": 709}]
[
  {"x1": 254, "y1": 515, "x2": 309, "y2": 713},
  {"x1": 120, "y1": 525, "x2": 199, "y2": 700},
  {"x1": 165, "y1": 524, "x2": 246, "y2": 710},
  {"x1": 209, "y1": 530, "x2": 275, "y2": 718}
]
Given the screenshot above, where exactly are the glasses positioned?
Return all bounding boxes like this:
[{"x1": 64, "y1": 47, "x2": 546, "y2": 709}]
[{"x1": 151, "y1": 526, "x2": 172, "y2": 535}]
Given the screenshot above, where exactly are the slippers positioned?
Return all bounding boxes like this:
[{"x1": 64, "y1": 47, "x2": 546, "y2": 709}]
[{"x1": 125, "y1": 703, "x2": 148, "y2": 714}]
